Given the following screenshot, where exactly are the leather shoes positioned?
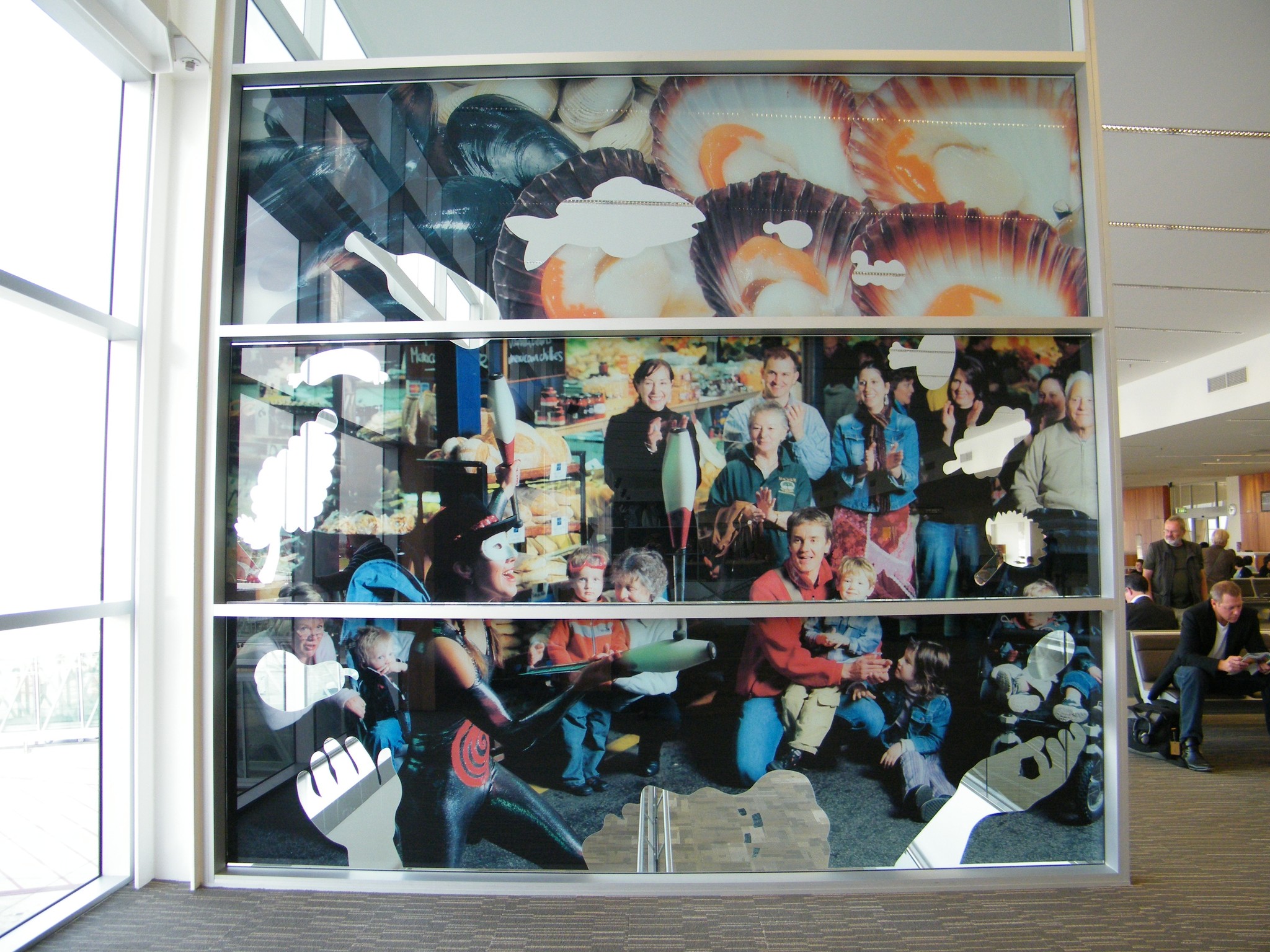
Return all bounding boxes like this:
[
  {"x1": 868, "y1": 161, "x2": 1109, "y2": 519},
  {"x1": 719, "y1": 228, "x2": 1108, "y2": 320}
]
[
  {"x1": 1181, "y1": 744, "x2": 1215, "y2": 771},
  {"x1": 640, "y1": 754, "x2": 660, "y2": 776}
]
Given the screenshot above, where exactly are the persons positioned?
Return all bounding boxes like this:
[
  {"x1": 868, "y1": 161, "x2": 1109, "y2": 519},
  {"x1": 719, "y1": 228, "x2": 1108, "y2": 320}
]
[
  {"x1": 236, "y1": 336, "x2": 1097, "y2": 867},
  {"x1": 1168, "y1": 580, "x2": 1270, "y2": 772},
  {"x1": 1123, "y1": 514, "x2": 1269, "y2": 630}
]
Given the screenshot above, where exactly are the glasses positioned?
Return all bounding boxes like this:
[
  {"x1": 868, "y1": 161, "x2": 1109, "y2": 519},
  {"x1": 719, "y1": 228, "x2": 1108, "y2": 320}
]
[
  {"x1": 568, "y1": 555, "x2": 607, "y2": 574},
  {"x1": 1162, "y1": 529, "x2": 1178, "y2": 534},
  {"x1": 294, "y1": 629, "x2": 324, "y2": 640},
  {"x1": 1224, "y1": 601, "x2": 1243, "y2": 609}
]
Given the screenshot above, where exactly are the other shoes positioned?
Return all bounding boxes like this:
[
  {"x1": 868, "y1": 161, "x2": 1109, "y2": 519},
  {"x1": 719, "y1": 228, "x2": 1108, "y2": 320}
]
[
  {"x1": 1008, "y1": 691, "x2": 1042, "y2": 713},
  {"x1": 903, "y1": 784, "x2": 933, "y2": 819},
  {"x1": 802, "y1": 751, "x2": 838, "y2": 773},
  {"x1": 567, "y1": 783, "x2": 593, "y2": 796},
  {"x1": 766, "y1": 750, "x2": 804, "y2": 772},
  {"x1": 1053, "y1": 699, "x2": 1088, "y2": 723},
  {"x1": 588, "y1": 776, "x2": 608, "y2": 792},
  {"x1": 919, "y1": 794, "x2": 952, "y2": 823}
]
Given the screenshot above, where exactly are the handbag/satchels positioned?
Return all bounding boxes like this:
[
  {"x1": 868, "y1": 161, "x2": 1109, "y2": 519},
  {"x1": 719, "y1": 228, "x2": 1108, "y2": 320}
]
[{"x1": 1127, "y1": 702, "x2": 1182, "y2": 763}]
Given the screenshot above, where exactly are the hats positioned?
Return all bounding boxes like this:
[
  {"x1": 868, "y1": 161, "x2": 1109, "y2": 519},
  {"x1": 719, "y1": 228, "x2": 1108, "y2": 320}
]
[{"x1": 421, "y1": 493, "x2": 519, "y2": 565}]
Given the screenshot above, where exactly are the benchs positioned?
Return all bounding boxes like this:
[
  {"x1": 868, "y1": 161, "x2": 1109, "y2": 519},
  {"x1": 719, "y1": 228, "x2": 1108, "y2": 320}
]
[
  {"x1": 1126, "y1": 630, "x2": 1270, "y2": 769},
  {"x1": 1230, "y1": 578, "x2": 1270, "y2": 604}
]
